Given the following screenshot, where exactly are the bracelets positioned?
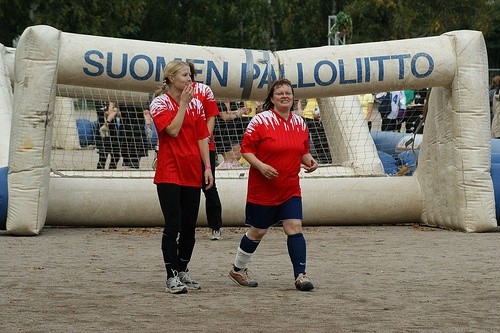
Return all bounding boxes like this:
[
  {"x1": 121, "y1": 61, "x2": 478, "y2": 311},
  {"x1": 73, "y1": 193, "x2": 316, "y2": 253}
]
[{"x1": 205, "y1": 164, "x2": 210, "y2": 170}]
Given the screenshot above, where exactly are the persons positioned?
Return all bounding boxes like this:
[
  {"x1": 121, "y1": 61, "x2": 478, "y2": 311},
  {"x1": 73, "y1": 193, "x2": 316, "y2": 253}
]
[
  {"x1": 229, "y1": 79, "x2": 320, "y2": 290},
  {"x1": 94, "y1": 59, "x2": 436, "y2": 292}
]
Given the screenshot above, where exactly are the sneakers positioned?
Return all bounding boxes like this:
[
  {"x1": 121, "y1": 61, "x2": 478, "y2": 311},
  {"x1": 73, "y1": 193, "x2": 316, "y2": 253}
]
[
  {"x1": 294, "y1": 273, "x2": 314, "y2": 290},
  {"x1": 210, "y1": 230, "x2": 222, "y2": 240},
  {"x1": 228, "y1": 264, "x2": 258, "y2": 287},
  {"x1": 165, "y1": 269, "x2": 201, "y2": 294}
]
